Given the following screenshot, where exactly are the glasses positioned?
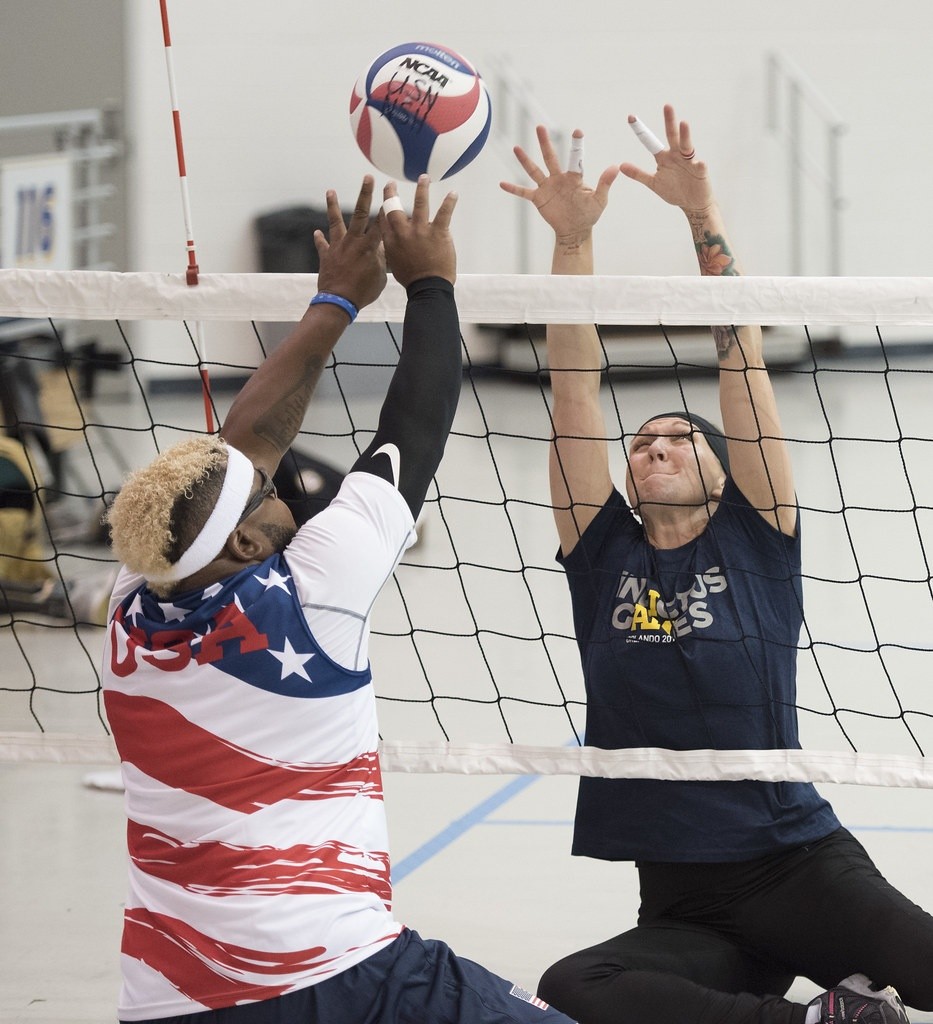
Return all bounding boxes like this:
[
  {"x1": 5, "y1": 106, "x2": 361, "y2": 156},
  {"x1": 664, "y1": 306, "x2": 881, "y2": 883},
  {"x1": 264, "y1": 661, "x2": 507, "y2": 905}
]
[{"x1": 233, "y1": 466, "x2": 278, "y2": 530}]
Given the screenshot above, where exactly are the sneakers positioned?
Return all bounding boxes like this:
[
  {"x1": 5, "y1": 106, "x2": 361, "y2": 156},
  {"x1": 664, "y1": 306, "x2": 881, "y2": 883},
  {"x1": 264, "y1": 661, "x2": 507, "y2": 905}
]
[{"x1": 808, "y1": 973, "x2": 910, "y2": 1024}]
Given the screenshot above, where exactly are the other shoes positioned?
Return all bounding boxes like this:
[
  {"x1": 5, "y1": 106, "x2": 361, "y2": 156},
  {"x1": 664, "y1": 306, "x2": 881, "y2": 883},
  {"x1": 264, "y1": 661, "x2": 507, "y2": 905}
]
[{"x1": 66, "y1": 569, "x2": 118, "y2": 627}]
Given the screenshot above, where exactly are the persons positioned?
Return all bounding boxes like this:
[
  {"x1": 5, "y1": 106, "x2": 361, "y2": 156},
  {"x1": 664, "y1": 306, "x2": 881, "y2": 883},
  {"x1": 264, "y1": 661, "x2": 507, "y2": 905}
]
[
  {"x1": 100, "y1": 174, "x2": 585, "y2": 1023},
  {"x1": 496, "y1": 99, "x2": 933, "y2": 1024},
  {"x1": 5, "y1": 344, "x2": 89, "y2": 500}
]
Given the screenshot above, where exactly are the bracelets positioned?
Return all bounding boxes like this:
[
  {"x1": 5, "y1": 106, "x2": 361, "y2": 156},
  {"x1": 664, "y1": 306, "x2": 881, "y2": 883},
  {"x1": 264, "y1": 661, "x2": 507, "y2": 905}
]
[{"x1": 306, "y1": 288, "x2": 359, "y2": 323}]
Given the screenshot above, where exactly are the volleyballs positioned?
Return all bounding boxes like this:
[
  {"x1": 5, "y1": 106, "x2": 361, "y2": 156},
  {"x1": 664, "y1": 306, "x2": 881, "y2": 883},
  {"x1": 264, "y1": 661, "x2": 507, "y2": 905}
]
[{"x1": 349, "y1": 40, "x2": 498, "y2": 187}]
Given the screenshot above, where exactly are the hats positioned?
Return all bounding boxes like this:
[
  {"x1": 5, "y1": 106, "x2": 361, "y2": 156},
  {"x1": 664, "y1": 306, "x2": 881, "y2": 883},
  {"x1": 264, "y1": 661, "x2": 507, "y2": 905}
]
[{"x1": 632, "y1": 412, "x2": 731, "y2": 476}]
